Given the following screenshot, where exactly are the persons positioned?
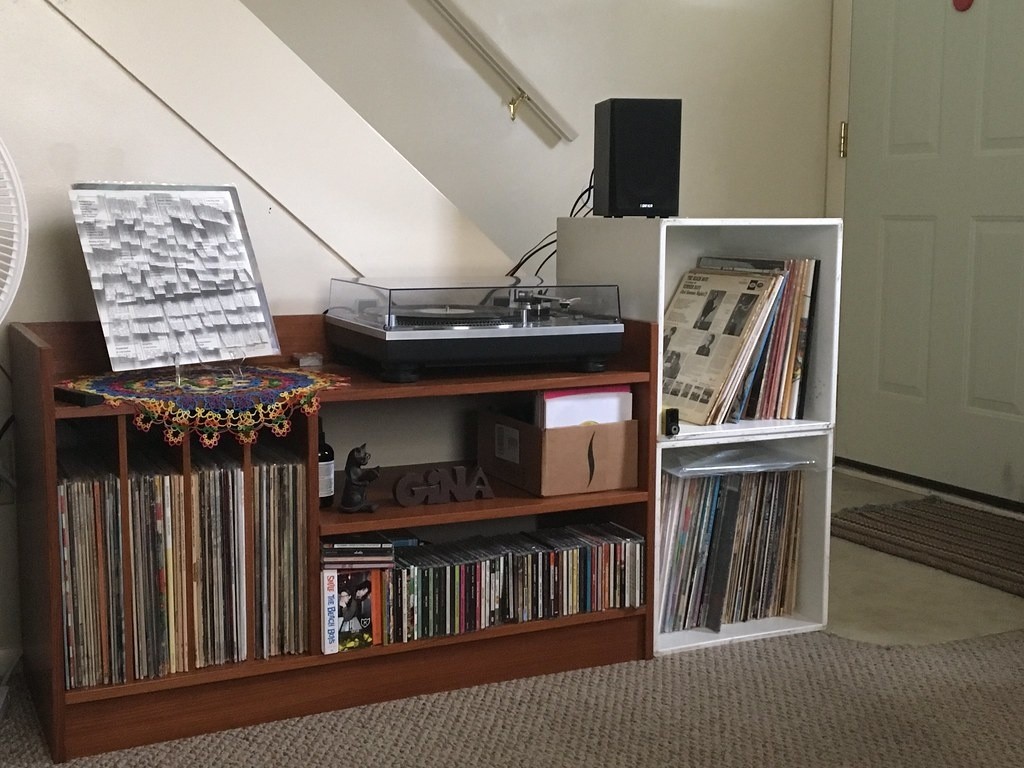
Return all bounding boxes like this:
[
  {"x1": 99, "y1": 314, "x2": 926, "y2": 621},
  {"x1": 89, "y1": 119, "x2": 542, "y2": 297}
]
[{"x1": 339, "y1": 587, "x2": 358, "y2": 622}]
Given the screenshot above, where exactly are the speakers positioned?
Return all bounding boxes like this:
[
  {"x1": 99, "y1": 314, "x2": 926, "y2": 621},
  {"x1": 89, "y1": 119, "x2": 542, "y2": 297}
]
[{"x1": 592, "y1": 98, "x2": 682, "y2": 217}]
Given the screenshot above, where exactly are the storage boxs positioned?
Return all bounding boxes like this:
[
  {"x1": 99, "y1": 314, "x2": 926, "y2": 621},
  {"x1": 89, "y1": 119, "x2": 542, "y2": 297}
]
[{"x1": 475, "y1": 408, "x2": 639, "y2": 498}]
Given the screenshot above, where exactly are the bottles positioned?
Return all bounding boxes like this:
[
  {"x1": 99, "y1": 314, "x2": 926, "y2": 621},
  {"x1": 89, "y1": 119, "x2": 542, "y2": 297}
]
[{"x1": 318, "y1": 417, "x2": 335, "y2": 508}]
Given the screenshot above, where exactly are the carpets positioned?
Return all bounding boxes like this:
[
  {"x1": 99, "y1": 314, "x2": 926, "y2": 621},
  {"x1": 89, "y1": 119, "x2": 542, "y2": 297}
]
[{"x1": 831, "y1": 495, "x2": 1024, "y2": 599}]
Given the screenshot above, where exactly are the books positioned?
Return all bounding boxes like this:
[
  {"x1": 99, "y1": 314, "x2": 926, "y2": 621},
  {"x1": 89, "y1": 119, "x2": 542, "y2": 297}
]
[
  {"x1": 661, "y1": 256, "x2": 822, "y2": 634},
  {"x1": 56, "y1": 450, "x2": 645, "y2": 690}
]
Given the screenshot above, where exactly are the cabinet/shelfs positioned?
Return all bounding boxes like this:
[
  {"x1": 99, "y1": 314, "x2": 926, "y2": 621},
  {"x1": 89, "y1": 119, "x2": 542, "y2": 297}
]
[
  {"x1": 9, "y1": 313, "x2": 659, "y2": 764},
  {"x1": 555, "y1": 216, "x2": 843, "y2": 658}
]
